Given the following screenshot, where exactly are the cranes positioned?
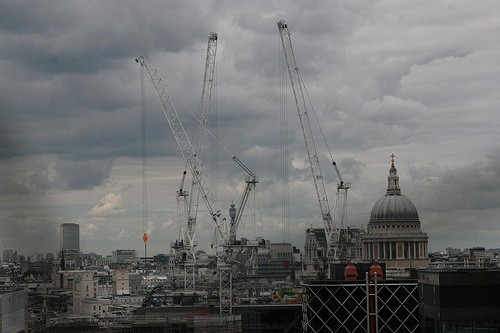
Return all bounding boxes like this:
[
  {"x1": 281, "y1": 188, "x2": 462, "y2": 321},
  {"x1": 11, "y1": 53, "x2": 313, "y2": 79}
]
[
  {"x1": 272, "y1": 19, "x2": 349, "y2": 281},
  {"x1": 134, "y1": 54, "x2": 263, "y2": 287},
  {"x1": 164, "y1": 32, "x2": 221, "y2": 283}
]
